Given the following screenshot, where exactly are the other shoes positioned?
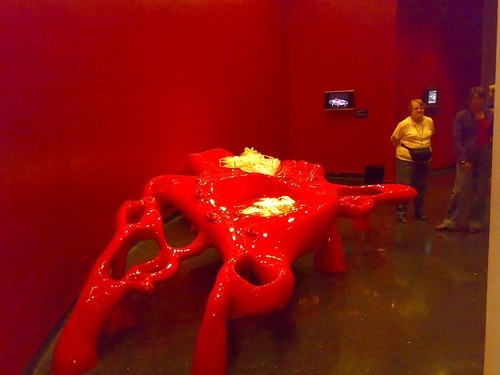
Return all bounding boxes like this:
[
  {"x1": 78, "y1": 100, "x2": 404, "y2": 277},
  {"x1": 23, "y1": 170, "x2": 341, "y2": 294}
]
[
  {"x1": 396, "y1": 211, "x2": 429, "y2": 225},
  {"x1": 435, "y1": 224, "x2": 479, "y2": 233}
]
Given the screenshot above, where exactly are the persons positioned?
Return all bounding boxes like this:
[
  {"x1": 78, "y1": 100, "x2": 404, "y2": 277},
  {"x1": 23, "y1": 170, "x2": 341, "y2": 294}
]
[
  {"x1": 436, "y1": 87, "x2": 493, "y2": 234},
  {"x1": 390, "y1": 99, "x2": 434, "y2": 223}
]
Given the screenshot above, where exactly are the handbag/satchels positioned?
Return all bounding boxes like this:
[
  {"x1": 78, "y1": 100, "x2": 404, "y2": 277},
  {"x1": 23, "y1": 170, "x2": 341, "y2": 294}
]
[{"x1": 408, "y1": 147, "x2": 432, "y2": 162}]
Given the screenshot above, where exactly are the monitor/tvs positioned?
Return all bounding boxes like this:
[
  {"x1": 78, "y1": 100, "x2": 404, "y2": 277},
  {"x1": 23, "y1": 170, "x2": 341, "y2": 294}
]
[
  {"x1": 423, "y1": 87, "x2": 438, "y2": 107},
  {"x1": 323, "y1": 90, "x2": 354, "y2": 111}
]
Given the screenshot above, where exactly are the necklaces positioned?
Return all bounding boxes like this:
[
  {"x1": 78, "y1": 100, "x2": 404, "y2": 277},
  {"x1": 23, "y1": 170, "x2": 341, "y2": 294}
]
[{"x1": 414, "y1": 124, "x2": 423, "y2": 145}]
